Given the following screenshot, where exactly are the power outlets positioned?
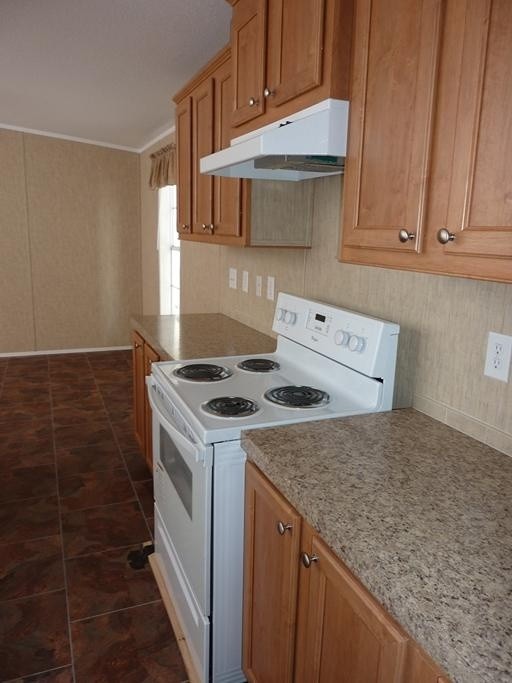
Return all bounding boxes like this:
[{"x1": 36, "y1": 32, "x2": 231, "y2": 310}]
[{"x1": 484, "y1": 332, "x2": 512, "y2": 383}]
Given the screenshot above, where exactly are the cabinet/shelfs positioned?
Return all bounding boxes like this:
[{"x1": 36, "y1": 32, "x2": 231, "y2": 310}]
[
  {"x1": 226, "y1": 0, "x2": 354, "y2": 143},
  {"x1": 172, "y1": 42, "x2": 313, "y2": 248},
  {"x1": 241, "y1": 460, "x2": 452, "y2": 683},
  {"x1": 130, "y1": 329, "x2": 159, "y2": 475},
  {"x1": 337, "y1": 0, "x2": 512, "y2": 284}
]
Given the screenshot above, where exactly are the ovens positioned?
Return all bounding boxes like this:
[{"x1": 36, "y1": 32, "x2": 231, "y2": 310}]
[{"x1": 142, "y1": 374, "x2": 244, "y2": 681}]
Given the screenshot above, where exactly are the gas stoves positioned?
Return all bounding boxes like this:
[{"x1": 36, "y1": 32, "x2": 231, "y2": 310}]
[{"x1": 150, "y1": 335, "x2": 382, "y2": 445}]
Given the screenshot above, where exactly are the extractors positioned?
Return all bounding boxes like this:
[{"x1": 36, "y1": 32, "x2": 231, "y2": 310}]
[{"x1": 200, "y1": 96, "x2": 351, "y2": 184}]
